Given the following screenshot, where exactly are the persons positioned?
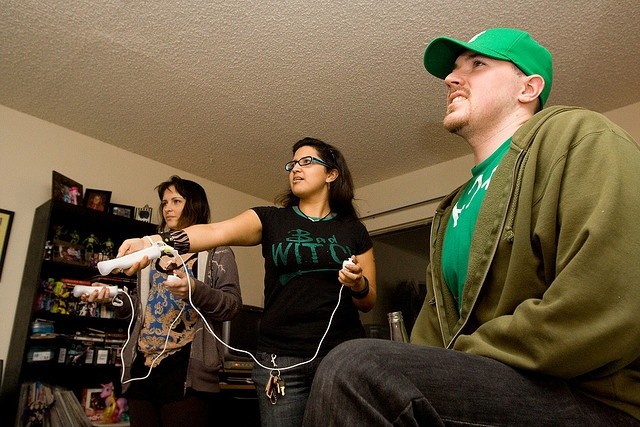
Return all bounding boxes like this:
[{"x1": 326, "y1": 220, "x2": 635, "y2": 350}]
[
  {"x1": 300, "y1": 25, "x2": 639, "y2": 426},
  {"x1": 89, "y1": 193, "x2": 103, "y2": 208},
  {"x1": 52, "y1": 224, "x2": 115, "y2": 255},
  {"x1": 112, "y1": 136, "x2": 378, "y2": 426},
  {"x1": 81, "y1": 175, "x2": 243, "y2": 426}
]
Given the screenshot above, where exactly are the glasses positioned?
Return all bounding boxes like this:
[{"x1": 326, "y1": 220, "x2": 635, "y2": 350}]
[{"x1": 285, "y1": 156, "x2": 333, "y2": 170}]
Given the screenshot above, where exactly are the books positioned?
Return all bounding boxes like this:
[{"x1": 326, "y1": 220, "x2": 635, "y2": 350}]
[
  {"x1": 60, "y1": 279, "x2": 92, "y2": 286},
  {"x1": 18, "y1": 381, "x2": 89, "y2": 427}
]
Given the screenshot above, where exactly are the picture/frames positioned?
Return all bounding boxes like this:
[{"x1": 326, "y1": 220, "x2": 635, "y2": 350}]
[
  {"x1": 0, "y1": 209, "x2": 14, "y2": 280},
  {"x1": 112, "y1": 203, "x2": 135, "y2": 217},
  {"x1": 85, "y1": 189, "x2": 112, "y2": 212},
  {"x1": 52, "y1": 170, "x2": 84, "y2": 204}
]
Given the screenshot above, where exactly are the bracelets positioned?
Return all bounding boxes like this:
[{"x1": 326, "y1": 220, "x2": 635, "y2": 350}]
[
  {"x1": 348, "y1": 275, "x2": 370, "y2": 299},
  {"x1": 169, "y1": 230, "x2": 191, "y2": 256},
  {"x1": 158, "y1": 230, "x2": 175, "y2": 249}
]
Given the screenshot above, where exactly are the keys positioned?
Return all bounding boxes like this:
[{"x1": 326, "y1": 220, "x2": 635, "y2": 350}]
[{"x1": 263, "y1": 364, "x2": 286, "y2": 405}]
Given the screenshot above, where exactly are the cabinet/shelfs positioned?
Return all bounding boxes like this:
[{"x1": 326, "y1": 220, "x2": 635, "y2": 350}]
[{"x1": 2, "y1": 199, "x2": 160, "y2": 426}]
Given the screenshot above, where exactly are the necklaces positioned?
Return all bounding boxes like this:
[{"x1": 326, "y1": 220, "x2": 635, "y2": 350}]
[{"x1": 296, "y1": 202, "x2": 333, "y2": 223}]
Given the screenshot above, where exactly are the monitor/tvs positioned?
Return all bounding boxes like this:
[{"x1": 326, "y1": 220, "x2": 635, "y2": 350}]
[{"x1": 222, "y1": 304, "x2": 264, "y2": 361}]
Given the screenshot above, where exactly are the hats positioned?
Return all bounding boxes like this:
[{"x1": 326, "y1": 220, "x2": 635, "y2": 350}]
[{"x1": 423, "y1": 28, "x2": 553, "y2": 109}]
